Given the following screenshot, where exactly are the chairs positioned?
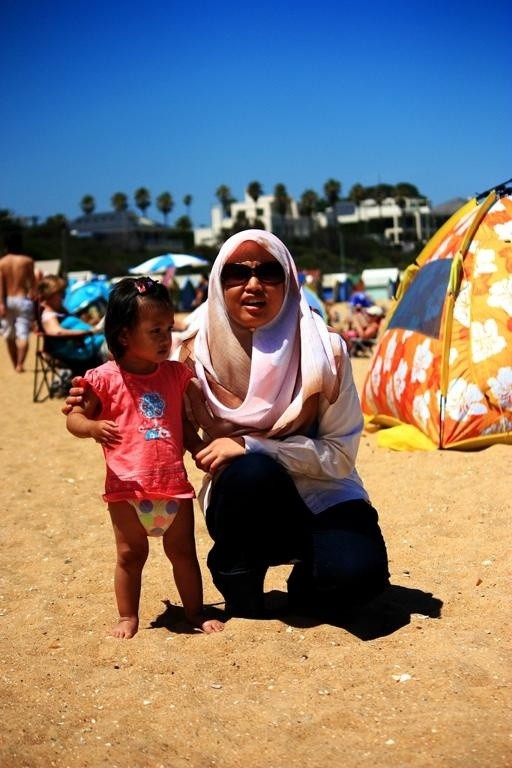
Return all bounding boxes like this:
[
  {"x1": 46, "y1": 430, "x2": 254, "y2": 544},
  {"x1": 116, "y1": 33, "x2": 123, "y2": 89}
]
[{"x1": 31, "y1": 302, "x2": 100, "y2": 403}]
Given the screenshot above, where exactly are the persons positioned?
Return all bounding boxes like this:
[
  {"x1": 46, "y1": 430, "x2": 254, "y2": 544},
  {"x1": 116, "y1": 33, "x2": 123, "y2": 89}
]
[
  {"x1": 66, "y1": 275, "x2": 226, "y2": 639},
  {"x1": 62, "y1": 229, "x2": 387, "y2": 625},
  {"x1": 1, "y1": 235, "x2": 387, "y2": 398}
]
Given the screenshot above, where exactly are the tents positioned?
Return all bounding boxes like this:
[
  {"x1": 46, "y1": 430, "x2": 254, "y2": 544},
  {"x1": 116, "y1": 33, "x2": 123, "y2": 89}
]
[{"x1": 361, "y1": 178, "x2": 512, "y2": 451}]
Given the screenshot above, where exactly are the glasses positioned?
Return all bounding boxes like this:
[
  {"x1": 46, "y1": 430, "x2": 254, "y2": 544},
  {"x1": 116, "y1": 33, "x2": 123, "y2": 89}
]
[{"x1": 220, "y1": 261, "x2": 284, "y2": 288}]
